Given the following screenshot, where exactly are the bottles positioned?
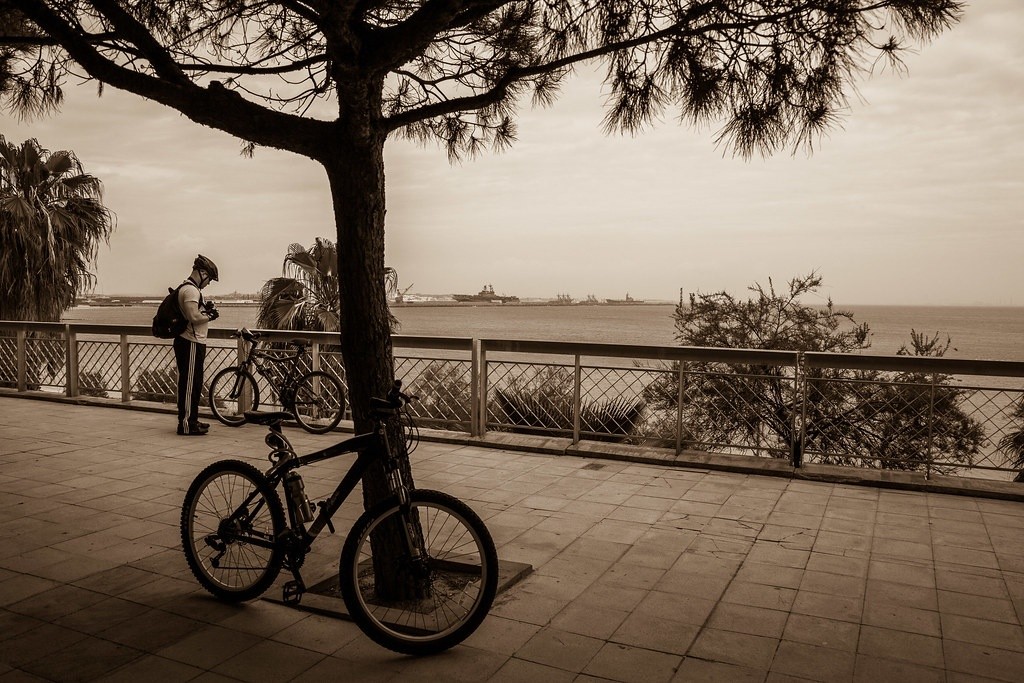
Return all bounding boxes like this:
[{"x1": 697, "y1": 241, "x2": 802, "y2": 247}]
[
  {"x1": 269, "y1": 370, "x2": 283, "y2": 391},
  {"x1": 285, "y1": 472, "x2": 313, "y2": 523}
]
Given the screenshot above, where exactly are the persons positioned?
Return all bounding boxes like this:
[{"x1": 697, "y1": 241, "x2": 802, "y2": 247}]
[{"x1": 172, "y1": 252, "x2": 220, "y2": 437}]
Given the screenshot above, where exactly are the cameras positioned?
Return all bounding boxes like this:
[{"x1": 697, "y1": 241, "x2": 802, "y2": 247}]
[{"x1": 205, "y1": 301, "x2": 215, "y2": 315}]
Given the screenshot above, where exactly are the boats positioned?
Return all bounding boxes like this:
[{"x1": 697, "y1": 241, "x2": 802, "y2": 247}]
[
  {"x1": 89, "y1": 304, "x2": 132, "y2": 307},
  {"x1": 451, "y1": 284, "x2": 520, "y2": 305},
  {"x1": 606, "y1": 292, "x2": 644, "y2": 305},
  {"x1": 579, "y1": 293, "x2": 598, "y2": 305},
  {"x1": 548, "y1": 293, "x2": 574, "y2": 305}
]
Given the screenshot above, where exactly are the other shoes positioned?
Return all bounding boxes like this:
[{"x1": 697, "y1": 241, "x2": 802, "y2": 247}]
[
  {"x1": 177, "y1": 425, "x2": 208, "y2": 435},
  {"x1": 197, "y1": 421, "x2": 210, "y2": 428}
]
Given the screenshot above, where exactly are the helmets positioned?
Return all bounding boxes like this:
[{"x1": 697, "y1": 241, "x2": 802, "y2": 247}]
[{"x1": 193, "y1": 254, "x2": 219, "y2": 285}]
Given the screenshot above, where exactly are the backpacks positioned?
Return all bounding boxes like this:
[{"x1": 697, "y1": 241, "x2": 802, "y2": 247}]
[{"x1": 152, "y1": 282, "x2": 203, "y2": 339}]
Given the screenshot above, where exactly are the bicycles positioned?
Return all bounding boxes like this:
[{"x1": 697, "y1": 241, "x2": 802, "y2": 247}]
[
  {"x1": 179, "y1": 380, "x2": 500, "y2": 657},
  {"x1": 208, "y1": 330, "x2": 347, "y2": 434}
]
